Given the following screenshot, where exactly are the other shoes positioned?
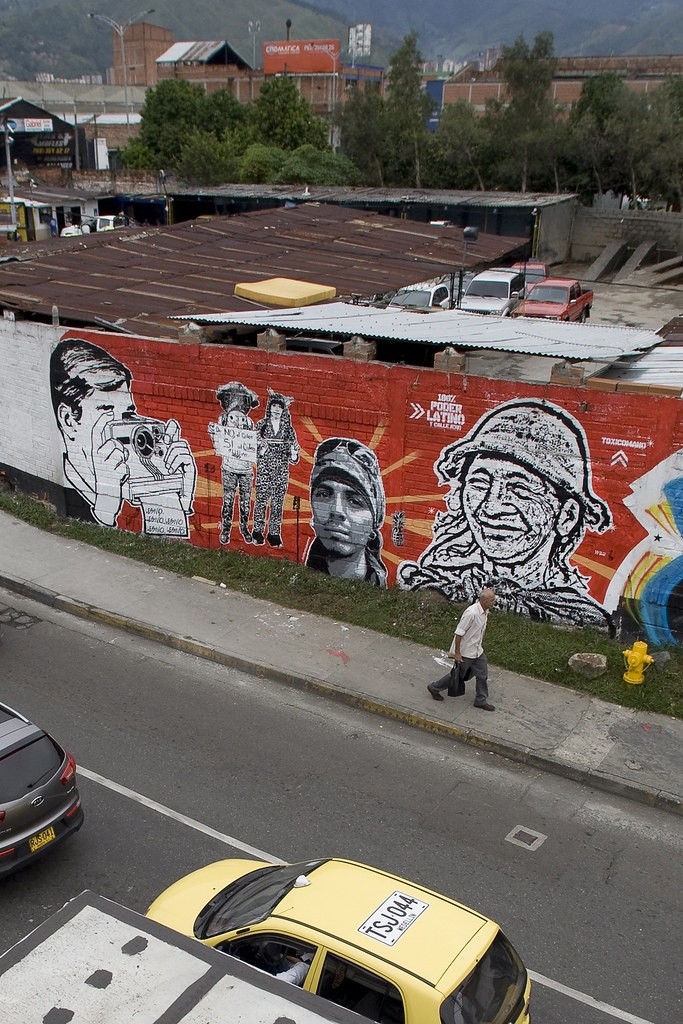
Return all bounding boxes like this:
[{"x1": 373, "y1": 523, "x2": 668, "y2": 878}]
[
  {"x1": 474, "y1": 703, "x2": 495, "y2": 711},
  {"x1": 427, "y1": 685, "x2": 444, "y2": 701}
]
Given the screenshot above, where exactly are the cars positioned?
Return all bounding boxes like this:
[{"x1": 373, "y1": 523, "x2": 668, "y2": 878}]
[
  {"x1": 60, "y1": 214, "x2": 125, "y2": 238},
  {"x1": 510, "y1": 262, "x2": 551, "y2": 293},
  {"x1": 384, "y1": 282, "x2": 451, "y2": 312}
]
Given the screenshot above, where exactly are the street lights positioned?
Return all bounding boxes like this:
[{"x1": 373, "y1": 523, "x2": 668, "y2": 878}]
[
  {"x1": 310, "y1": 41, "x2": 360, "y2": 147},
  {"x1": 247, "y1": 19, "x2": 262, "y2": 69},
  {"x1": 89, "y1": 8, "x2": 156, "y2": 137}
]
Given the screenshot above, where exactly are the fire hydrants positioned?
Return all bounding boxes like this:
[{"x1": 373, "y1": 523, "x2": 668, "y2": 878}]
[{"x1": 621, "y1": 642, "x2": 655, "y2": 685}]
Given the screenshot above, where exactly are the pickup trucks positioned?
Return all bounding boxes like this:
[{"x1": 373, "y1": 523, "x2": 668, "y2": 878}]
[{"x1": 514, "y1": 277, "x2": 593, "y2": 323}]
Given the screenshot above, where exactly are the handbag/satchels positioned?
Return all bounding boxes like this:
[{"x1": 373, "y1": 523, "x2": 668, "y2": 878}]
[{"x1": 448, "y1": 662, "x2": 465, "y2": 697}]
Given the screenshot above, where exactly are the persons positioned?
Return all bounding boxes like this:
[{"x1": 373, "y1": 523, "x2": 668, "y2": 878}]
[
  {"x1": 274, "y1": 944, "x2": 315, "y2": 986},
  {"x1": 426, "y1": 589, "x2": 495, "y2": 712}
]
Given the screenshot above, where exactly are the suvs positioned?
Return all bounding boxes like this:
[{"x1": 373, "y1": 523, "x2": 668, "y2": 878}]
[
  {"x1": 454, "y1": 267, "x2": 528, "y2": 318},
  {"x1": 0, "y1": 703, "x2": 84, "y2": 879},
  {"x1": 139, "y1": 857, "x2": 531, "y2": 1024}
]
